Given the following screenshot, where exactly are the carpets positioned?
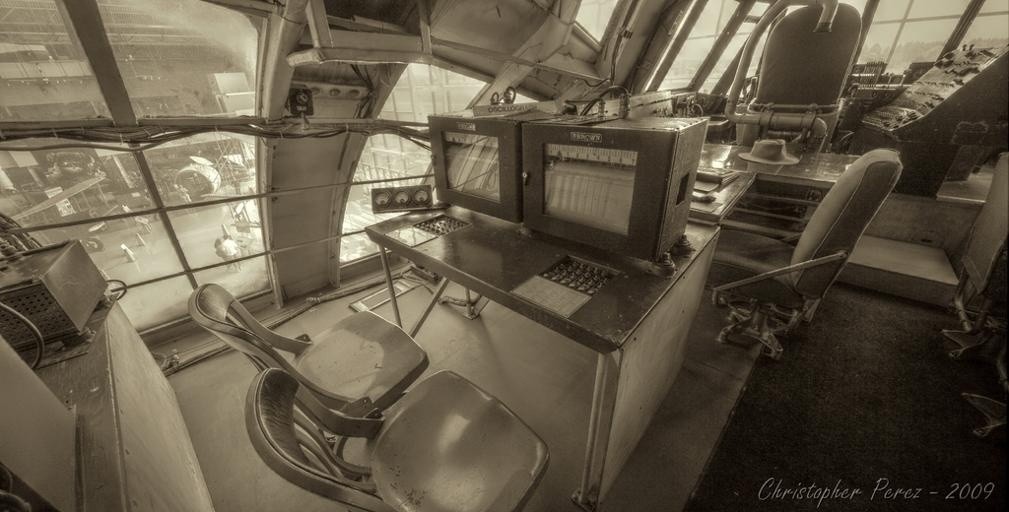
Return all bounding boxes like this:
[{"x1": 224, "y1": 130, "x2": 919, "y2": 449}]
[{"x1": 681, "y1": 282, "x2": 1009, "y2": 512}]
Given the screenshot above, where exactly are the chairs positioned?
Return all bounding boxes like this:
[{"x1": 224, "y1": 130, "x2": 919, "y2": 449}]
[
  {"x1": 188, "y1": 283, "x2": 551, "y2": 512},
  {"x1": 713, "y1": 148, "x2": 903, "y2": 362}
]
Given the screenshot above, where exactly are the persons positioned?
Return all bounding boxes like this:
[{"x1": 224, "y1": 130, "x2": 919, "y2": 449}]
[
  {"x1": 230, "y1": 177, "x2": 241, "y2": 194},
  {"x1": 214, "y1": 239, "x2": 233, "y2": 268},
  {"x1": 177, "y1": 184, "x2": 194, "y2": 205},
  {"x1": 222, "y1": 235, "x2": 240, "y2": 270}
]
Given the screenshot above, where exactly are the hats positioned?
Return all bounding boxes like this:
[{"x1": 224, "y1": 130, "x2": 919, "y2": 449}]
[{"x1": 739, "y1": 139, "x2": 799, "y2": 165}]
